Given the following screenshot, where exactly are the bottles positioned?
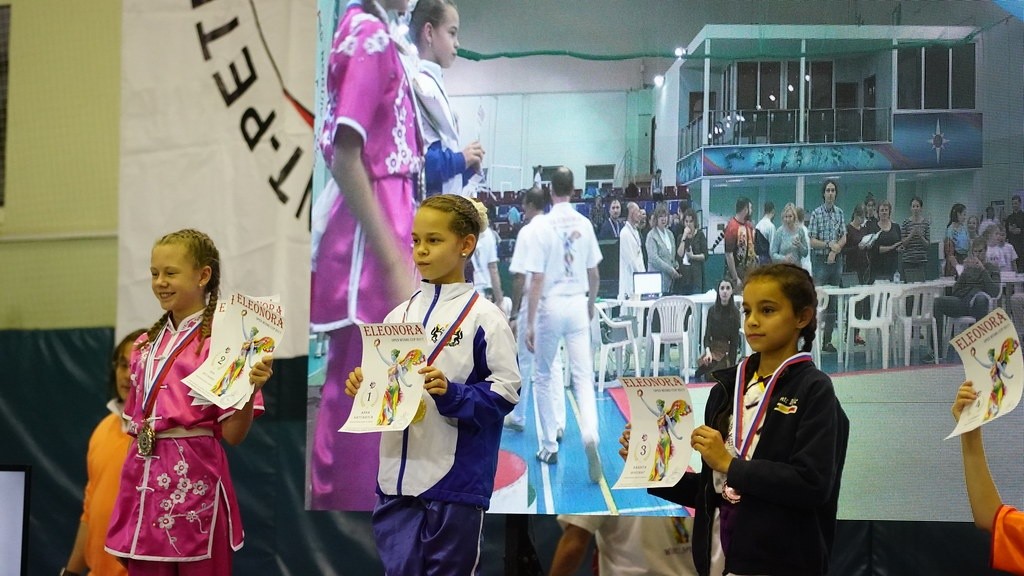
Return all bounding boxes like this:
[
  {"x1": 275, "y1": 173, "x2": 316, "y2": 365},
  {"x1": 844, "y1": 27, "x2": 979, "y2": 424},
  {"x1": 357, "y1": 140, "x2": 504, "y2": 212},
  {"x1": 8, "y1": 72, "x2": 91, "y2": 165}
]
[{"x1": 893, "y1": 269, "x2": 900, "y2": 284}]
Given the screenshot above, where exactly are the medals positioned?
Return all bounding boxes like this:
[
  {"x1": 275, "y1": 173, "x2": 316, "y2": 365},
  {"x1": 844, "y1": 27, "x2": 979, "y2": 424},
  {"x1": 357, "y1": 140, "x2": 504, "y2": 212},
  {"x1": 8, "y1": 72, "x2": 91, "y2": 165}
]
[
  {"x1": 721, "y1": 480, "x2": 744, "y2": 504},
  {"x1": 411, "y1": 398, "x2": 427, "y2": 424},
  {"x1": 137, "y1": 423, "x2": 155, "y2": 455}
]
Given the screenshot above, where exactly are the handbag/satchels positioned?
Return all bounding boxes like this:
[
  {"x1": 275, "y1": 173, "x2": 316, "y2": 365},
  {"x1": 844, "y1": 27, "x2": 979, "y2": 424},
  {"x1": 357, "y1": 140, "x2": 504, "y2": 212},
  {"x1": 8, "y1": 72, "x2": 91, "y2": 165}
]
[{"x1": 723, "y1": 257, "x2": 746, "y2": 292}]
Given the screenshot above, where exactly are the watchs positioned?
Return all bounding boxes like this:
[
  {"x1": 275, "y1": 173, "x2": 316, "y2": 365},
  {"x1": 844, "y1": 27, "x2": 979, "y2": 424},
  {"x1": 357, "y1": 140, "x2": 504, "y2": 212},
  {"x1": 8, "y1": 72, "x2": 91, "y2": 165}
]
[
  {"x1": 59, "y1": 566, "x2": 80, "y2": 576},
  {"x1": 825, "y1": 240, "x2": 830, "y2": 249}
]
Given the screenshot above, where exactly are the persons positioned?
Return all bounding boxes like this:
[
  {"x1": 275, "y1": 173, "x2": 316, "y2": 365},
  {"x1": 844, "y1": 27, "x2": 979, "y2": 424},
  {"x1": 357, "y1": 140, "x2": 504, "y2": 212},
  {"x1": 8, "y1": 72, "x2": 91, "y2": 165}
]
[
  {"x1": 309, "y1": 0, "x2": 1024, "y2": 509},
  {"x1": 952, "y1": 381, "x2": 1024, "y2": 576},
  {"x1": 639, "y1": 395, "x2": 682, "y2": 482},
  {"x1": 376, "y1": 345, "x2": 412, "y2": 426},
  {"x1": 66, "y1": 329, "x2": 151, "y2": 576},
  {"x1": 548, "y1": 514, "x2": 699, "y2": 576},
  {"x1": 619, "y1": 265, "x2": 850, "y2": 576},
  {"x1": 103, "y1": 228, "x2": 273, "y2": 576},
  {"x1": 974, "y1": 348, "x2": 1014, "y2": 422},
  {"x1": 345, "y1": 195, "x2": 522, "y2": 576}
]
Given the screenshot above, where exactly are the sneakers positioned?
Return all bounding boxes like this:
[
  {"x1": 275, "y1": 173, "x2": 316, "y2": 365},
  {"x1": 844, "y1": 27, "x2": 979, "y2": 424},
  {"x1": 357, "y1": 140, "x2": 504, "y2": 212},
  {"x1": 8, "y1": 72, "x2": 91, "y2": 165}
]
[{"x1": 921, "y1": 352, "x2": 943, "y2": 362}]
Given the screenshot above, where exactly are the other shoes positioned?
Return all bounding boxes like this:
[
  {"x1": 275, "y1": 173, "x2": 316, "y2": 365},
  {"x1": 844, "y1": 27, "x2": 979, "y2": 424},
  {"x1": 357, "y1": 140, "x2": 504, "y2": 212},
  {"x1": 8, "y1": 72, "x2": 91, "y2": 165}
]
[
  {"x1": 556, "y1": 429, "x2": 564, "y2": 444},
  {"x1": 911, "y1": 332, "x2": 924, "y2": 339},
  {"x1": 853, "y1": 335, "x2": 866, "y2": 345},
  {"x1": 585, "y1": 441, "x2": 602, "y2": 484},
  {"x1": 822, "y1": 343, "x2": 836, "y2": 352},
  {"x1": 535, "y1": 446, "x2": 557, "y2": 464},
  {"x1": 503, "y1": 413, "x2": 524, "y2": 431}
]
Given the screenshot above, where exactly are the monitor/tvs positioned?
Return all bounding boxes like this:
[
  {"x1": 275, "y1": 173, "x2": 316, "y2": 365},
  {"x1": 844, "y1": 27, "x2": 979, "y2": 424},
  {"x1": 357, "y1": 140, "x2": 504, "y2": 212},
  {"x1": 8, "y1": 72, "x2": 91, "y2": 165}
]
[{"x1": 634, "y1": 271, "x2": 663, "y2": 300}]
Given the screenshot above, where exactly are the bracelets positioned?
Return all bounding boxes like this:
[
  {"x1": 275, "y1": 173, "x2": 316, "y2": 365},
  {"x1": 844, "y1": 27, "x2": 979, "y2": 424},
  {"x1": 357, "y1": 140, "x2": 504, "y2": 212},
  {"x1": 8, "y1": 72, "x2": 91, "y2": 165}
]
[{"x1": 680, "y1": 238, "x2": 686, "y2": 242}]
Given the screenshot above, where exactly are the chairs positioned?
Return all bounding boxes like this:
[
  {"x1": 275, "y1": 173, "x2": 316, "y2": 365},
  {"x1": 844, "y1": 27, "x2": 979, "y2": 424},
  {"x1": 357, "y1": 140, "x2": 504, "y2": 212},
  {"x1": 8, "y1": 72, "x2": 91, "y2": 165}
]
[
  {"x1": 595, "y1": 280, "x2": 758, "y2": 393},
  {"x1": 796, "y1": 281, "x2": 1004, "y2": 370}
]
